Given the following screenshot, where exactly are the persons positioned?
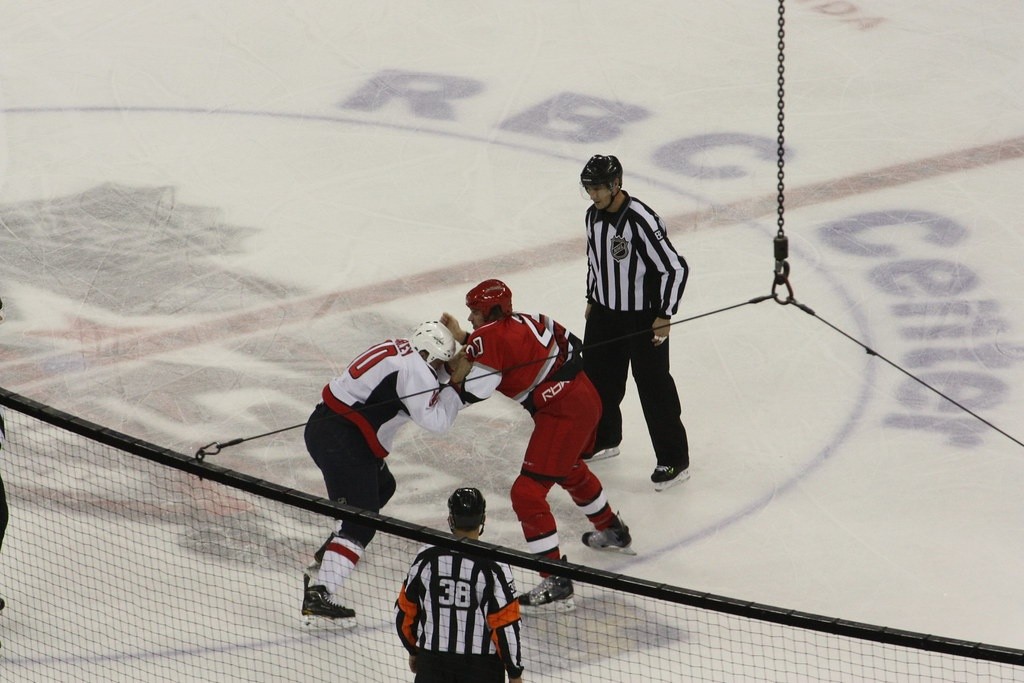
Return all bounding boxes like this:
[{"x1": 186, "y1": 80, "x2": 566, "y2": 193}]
[
  {"x1": 394, "y1": 487, "x2": 524, "y2": 683},
  {"x1": 439, "y1": 279, "x2": 637, "y2": 614},
  {"x1": 302, "y1": 320, "x2": 473, "y2": 632},
  {"x1": 581, "y1": 155, "x2": 689, "y2": 492}
]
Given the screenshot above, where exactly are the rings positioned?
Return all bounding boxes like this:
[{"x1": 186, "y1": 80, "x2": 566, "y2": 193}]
[{"x1": 655, "y1": 335, "x2": 667, "y2": 341}]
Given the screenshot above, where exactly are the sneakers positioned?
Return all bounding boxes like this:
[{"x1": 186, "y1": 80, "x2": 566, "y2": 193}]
[
  {"x1": 302, "y1": 570, "x2": 357, "y2": 632},
  {"x1": 651, "y1": 456, "x2": 691, "y2": 491},
  {"x1": 308, "y1": 532, "x2": 336, "y2": 569},
  {"x1": 514, "y1": 555, "x2": 576, "y2": 615},
  {"x1": 582, "y1": 510, "x2": 637, "y2": 555},
  {"x1": 581, "y1": 436, "x2": 622, "y2": 463}
]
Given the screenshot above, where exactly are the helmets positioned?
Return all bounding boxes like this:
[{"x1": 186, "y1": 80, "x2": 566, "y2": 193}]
[
  {"x1": 581, "y1": 154, "x2": 623, "y2": 192},
  {"x1": 411, "y1": 320, "x2": 456, "y2": 363},
  {"x1": 448, "y1": 487, "x2": 485, "y2": 515},
  {"x1": 466, "y1": 279, "x2": 513, "y2": 321}
]
